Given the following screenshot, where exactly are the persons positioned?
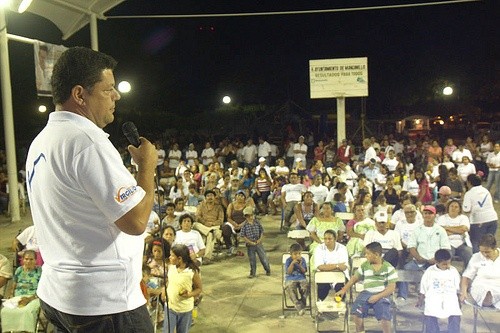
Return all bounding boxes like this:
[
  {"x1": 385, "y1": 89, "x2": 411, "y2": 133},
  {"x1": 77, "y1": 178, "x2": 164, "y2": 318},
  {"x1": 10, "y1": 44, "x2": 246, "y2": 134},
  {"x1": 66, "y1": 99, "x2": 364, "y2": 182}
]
[
  {"x1": 25, "y1": 46, "x2": 158, "y2": 333},
  {"x1": 0, "y1": 127, "x2": 500, "y2": 333},
  {"x1": 38, "y1": 45, "x2": 51, "y2": 89}
]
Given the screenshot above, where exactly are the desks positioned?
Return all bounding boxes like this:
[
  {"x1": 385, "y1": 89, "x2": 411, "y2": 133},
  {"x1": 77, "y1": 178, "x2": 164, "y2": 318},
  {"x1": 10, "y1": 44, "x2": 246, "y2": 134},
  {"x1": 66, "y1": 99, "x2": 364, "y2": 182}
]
[{"x1": 464, "y1": 293, "x2": 496, "y2": 333}]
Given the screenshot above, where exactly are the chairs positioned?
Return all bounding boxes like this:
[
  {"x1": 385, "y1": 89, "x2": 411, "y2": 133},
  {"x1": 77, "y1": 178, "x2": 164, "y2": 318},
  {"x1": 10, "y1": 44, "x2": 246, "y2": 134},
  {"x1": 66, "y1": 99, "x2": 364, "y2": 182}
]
[
  {"x1": 281, "y1": 253, "x2": 311, "y2": 314},
  {"x1": 349, "y1": 257, "x2": 394, "y2": 332},
  {"x1": 288, "y1": 230, "x2": 311, "y2": 257},
  {"x1": 396, "y1": 269, "x2": 427, "y2": 332},
  {"x1": 316, "y1": 269, "x2": 350, "y2": 332},
  {"x1": 336, "y1": 211, "x2": 354, "y2": 243}
]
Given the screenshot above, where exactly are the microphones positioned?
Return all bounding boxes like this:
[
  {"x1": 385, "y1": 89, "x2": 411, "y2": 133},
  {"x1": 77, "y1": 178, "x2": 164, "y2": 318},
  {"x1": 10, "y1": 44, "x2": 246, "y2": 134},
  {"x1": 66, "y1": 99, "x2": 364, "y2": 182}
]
[{"x1": 123, "y1": 122, "x2": 143, "y2": 149}]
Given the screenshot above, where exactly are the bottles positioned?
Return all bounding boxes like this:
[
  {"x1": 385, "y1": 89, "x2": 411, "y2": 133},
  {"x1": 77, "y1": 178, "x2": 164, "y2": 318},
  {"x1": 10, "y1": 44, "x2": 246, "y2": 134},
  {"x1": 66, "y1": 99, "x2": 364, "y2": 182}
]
[
  {"x1": 192, "y1": 307, "x2": 198, "y2": 322},
  {"x1": 335, "y1": 295, "x2": 342, "y2": 308}
]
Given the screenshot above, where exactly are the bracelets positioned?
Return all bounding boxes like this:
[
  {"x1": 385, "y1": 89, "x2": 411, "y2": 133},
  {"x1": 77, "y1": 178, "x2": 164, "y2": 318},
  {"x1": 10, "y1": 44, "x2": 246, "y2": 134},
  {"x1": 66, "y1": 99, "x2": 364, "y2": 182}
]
[
  {"x1": 33, "y1": 295, "x2": 37, "y2": 299},
  {"x1": 196, "y1": 254, "x2": 199, "y2": 258},
  {"x1": 336, "y1": 264, "x2": 340, "y2": 270}
]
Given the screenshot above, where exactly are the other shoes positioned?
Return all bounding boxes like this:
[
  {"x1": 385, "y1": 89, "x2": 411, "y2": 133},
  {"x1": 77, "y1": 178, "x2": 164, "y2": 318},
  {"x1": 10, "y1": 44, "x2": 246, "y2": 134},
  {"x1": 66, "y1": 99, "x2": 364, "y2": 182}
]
[
  {"x1": 202, "y1": 257, "x2": 211, "y2": 265},
  {"x1": 295, "y1": 301, "x2": 305, "y2": 316},
  {"x1": 226, "y1": 247, "x2": 234, "y2": 256},
  {"x1": 337, "y1": 312, "x2": 344, "y2": 316},
  {"x1": 216, "y1": 229, "x2": 222, "y2": 243}
]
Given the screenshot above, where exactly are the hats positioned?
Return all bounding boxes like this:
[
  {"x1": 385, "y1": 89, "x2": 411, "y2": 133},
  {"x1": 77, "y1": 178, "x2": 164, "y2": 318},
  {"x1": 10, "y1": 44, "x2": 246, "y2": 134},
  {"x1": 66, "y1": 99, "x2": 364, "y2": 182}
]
[
  {"x1": 259, "y1": 157, "x2": 266, "y2": 162},
  {"x1": 235, "y1": 190, "x2": 245, "y2": 196},
  {"x1": 437, "y1": 186, "x2": 451, "y2": 195},
  {"x1": 243, "y1": 207, "x2": 252, "y2": 216},
  {"x1": 374, "y1": 210, "x2": 388, "y2": 223},
  {"x1": 423, "y1": 205, "x2": 436, "y2": 213},
  {"x1": 296, "y1": 158, "x2": 303, "y2": 162}
]
